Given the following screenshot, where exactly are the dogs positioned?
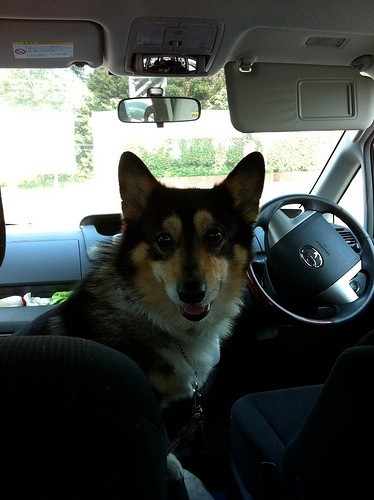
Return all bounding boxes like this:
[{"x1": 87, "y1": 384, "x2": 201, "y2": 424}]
[{"x1": 10, "y1": 150, "x2": 266, "y2": 406}]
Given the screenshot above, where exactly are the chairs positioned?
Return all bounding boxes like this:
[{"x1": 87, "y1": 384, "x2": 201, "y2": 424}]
[
  {"x1": 0, "y1": 334, "x2": 189, "y2": 500},
  {"x1": 226, "y1": 347, "x2": 374, "y2": 499}
]
